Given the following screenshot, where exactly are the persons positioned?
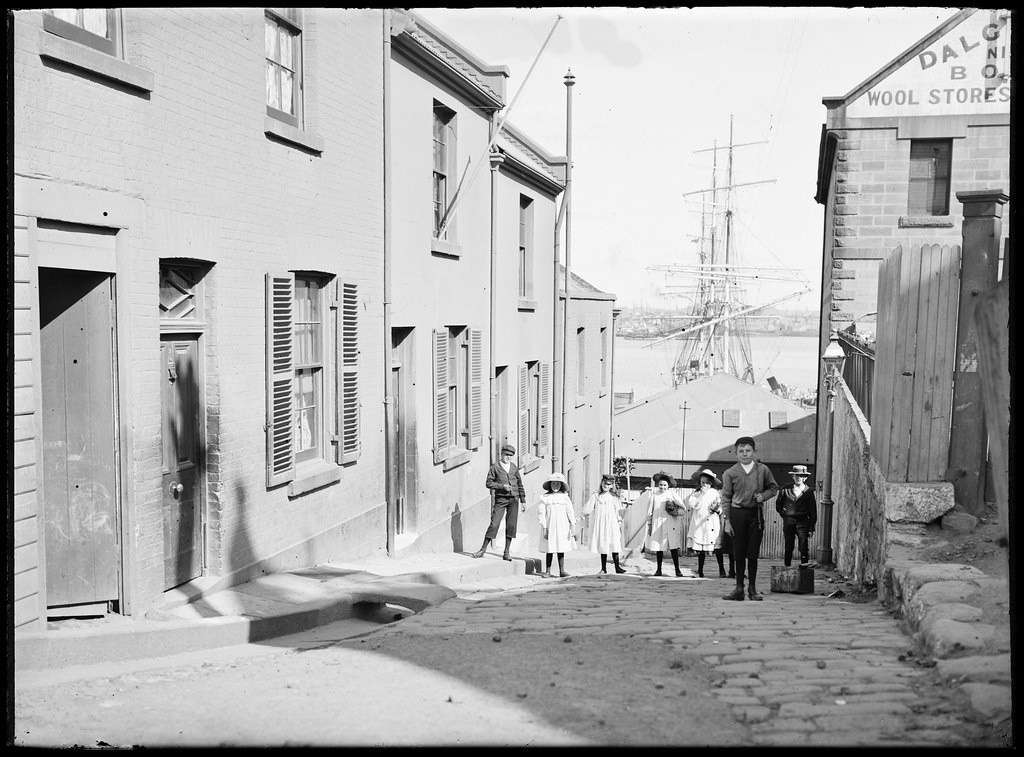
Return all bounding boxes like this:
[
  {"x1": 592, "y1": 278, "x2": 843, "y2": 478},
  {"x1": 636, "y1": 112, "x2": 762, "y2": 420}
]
[
  {"x1": 776, "y1": 465, "x2": 818, "y2": 566},
  {"x1": 687, "y1": 469, "x2": 726, "y2": 578},
  {"x1": 581, "y1": 474, "x2": 629, "y2": 574},
  {"x1": 471, "y1": 445, "x2": 527, "y2": 561},
  {"x1": 721, "y1": 437, "x2": 778, "y2": 600},
  {"x1": 645, "y1": 470, "x2": 688, "y2": 577},
  {"x1": 538, "y1": 473, "x2": 578, "y2": 578}
]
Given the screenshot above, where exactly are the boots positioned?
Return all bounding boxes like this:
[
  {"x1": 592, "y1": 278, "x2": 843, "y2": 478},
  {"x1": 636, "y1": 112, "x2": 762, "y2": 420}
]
[
  {"x1": 612, "y1": 552, "x2": 627, "y2": 573},
  {"x1": 670, "y1": 548, "x2": 684, "y2": 576},
  {"x1": 692, "y1": 552, "x2": 705, "y2": 577},
  {"x1": 542, "y1": 553, "x2": 553, "y2": 577},
  {"x1": 748, "y1": 557, "x2": 763, "y2": 600},
  {"x1": 557, "y1": 553, "x2": 571, "y2": 577},
  {"x1": 654, "y1": 550, "x2": 663, "y2": 575},
  {"x1": 715, "y1": 551, "x2": 727, "y2": 577},
  {"x1": 726, "y1": 553, "x2": 735, "y2": 578},
  {"x1": 721, "y1": 562, "x2": 745, "y2": 600},
  {"x1": 599, "y1": 553, "x2": 607, "y2": 574}
]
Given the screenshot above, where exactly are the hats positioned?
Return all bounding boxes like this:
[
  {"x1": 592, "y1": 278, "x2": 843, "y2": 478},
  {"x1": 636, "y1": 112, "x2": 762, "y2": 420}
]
[
  {"x1": 602, "y1": 474, "x2": 614, "y2": 481},
  {"x1": 691, "y1": 468, "x2": 722, "y2": 486},
  {"x1": 788, "y1": 465, "x2": 811, "y2": 475},
  {"x1": 502, "y1": 445, "x2": 515, "y2": 454},
  {"x1": 543, "y1": 472, "x2": 570, "y2": 492},
  {"x1": 653, "y1": 470, "x2": 677, "y2": 488}
]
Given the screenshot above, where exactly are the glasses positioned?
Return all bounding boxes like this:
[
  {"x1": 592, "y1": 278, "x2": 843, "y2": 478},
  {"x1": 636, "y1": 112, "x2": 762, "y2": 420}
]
[{"x1": 503, "y1": 452, "x2": 513, "y2": 456}]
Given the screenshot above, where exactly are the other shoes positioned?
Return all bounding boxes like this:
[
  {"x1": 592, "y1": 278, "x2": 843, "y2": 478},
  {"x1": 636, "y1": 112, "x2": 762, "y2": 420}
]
[
  {"x1": 503, "y1": 548, "x2": 512, "y2": 561},
  {"x1": 472, "y1": 547, "x2": 485, "y2": 558}
]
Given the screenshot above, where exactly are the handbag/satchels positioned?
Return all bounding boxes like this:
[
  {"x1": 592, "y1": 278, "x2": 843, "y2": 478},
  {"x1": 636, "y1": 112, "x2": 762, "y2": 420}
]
[{"x1": 666, "y1": 500, "x2": 676, "y2": 510}]
